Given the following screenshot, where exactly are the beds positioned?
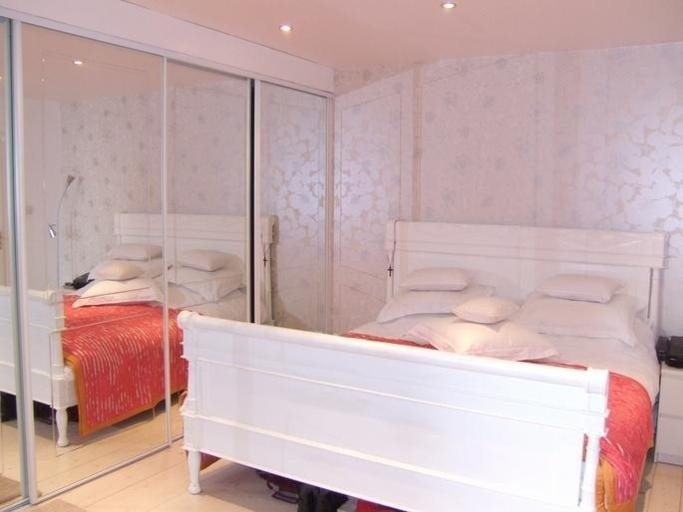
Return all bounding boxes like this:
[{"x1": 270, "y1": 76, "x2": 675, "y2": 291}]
[
  {"x1": 2, "y1": 212, "x2": 277, "y2": 447},
  {"x1": 177, "y1": 218, "x2": 677, "y2": 512}
]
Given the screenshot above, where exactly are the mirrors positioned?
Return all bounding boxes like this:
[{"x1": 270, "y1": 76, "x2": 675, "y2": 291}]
[{"x1": 1, "y1": 5, "x2": 334, "y2": 511}]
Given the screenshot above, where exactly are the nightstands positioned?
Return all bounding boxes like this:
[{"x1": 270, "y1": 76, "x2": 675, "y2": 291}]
[{"x1": 653, "y1": 362, "x2": 683, "y2": 467}]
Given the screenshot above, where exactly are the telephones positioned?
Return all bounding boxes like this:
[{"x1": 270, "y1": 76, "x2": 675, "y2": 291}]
[
  {"x1": 657, "y1": 336, "x2": 683, "y2": 368},
  {"x1": 65, "y1": 271, "x2": 92, "y2": 289}
]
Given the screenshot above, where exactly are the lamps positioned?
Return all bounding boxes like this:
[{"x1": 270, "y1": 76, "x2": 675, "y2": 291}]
[{"x1": 47, "y1": 175, "x2": 76, "y2": 291}]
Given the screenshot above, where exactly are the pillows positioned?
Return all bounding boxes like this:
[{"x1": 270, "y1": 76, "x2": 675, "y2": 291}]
[
  {"x1": 72, "y1": 241, "x2": 247, "y2": 312},
  {"x1": 374, "y1": 266, "x2": 650, "y2": 362}
]
